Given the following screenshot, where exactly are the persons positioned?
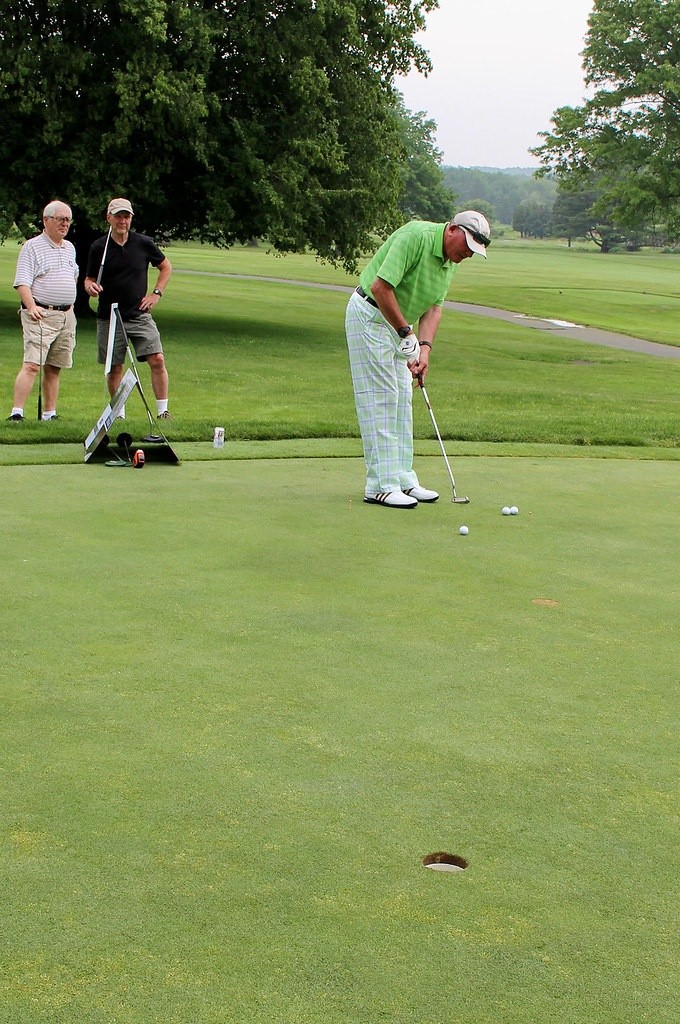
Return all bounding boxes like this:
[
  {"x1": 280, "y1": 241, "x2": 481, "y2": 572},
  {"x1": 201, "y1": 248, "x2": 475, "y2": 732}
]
[
  {"x1": 5, "y1": 201, "x2": 80, "y2": 422},
  {"x1": 84, "y1": 198, "x2": 173, "y2": 419},
  {"x1": 345, "y1": 209, "x2": 492, "y2": 509}
]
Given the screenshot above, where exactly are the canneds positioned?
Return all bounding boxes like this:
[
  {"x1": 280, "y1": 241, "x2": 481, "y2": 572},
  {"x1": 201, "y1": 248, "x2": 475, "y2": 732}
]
[{"x1": 213, "y1": 427, "x2": 225, "y2": 448}]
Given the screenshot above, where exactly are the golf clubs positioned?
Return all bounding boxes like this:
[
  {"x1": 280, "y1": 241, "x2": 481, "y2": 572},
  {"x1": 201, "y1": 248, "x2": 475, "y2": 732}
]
[
  {"x1": 113, "y1": 302, "x2": 159, "y2": 438},
  {"x1": 418, "y1": 372, "x2": 469, "y2": 504},
  {"x1": 95, "y1": 224, "x2": 113, "y2": 293},
  {"x1": 37, "y1": 316, "x2": 46, "y2": 420}
]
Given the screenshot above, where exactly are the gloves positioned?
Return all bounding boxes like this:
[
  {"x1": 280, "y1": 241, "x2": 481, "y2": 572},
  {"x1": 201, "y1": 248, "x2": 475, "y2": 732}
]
[{"x1": 398, "y1": 333, "x2": 421, "y2": 364}]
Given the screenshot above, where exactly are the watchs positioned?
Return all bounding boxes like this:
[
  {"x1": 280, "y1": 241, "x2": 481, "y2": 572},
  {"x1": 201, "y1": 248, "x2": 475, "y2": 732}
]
[
  {"x1": 153, "y1": 289, "x2": 161, "y2": 298},
  {"x1": 398, "y1": 327, "x2": 410, "y2": 338}
]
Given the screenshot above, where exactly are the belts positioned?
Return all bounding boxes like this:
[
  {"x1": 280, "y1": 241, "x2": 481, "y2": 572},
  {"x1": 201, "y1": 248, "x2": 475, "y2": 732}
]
[
  {"x1": 35, "y1": 301, "x2": 70, "y2": 311},
  {"x1": 356, "y1": 285, "x2": 379, "y2": 310}
]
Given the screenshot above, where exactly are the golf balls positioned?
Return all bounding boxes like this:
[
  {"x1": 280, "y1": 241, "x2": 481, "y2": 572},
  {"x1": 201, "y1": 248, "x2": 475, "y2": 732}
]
[
  {"x1": 460, "y1": 526, "x2": 468, "y2": 535},
  {"x1": 501, "y1": 506, "x2": 510, "y2": 515},
  {"x1": 510, "y1": 506, "x2": 518, "y2": 515}
]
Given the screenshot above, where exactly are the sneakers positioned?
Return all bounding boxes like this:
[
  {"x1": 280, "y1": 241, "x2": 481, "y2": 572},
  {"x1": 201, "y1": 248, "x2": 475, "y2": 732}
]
[
  {"x1": 5, "y1": 414, "x2": 26, "y2": 423},
  {"x1": 363, "y1": 488, "x2": 418, "y2": 508},
  {"x1": 402, "y1": 487, "x2": 439, "y2": 502},
  {"x1": 156, "y1": 411, "x2": 176, "y2": 421},
  {"x1": 41, "y1": 415, "x2": 66, "y2": 422}
]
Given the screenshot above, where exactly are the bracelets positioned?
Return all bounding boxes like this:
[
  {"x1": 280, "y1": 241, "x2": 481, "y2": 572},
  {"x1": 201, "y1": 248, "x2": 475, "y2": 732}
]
[{"x1": 419, "y1": 341, "x2": 432, "y2": 348}]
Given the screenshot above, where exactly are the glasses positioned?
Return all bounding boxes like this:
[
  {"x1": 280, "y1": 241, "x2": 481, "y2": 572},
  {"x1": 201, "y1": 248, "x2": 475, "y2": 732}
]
[
  {"x1": 458, "y1": 224, "x2": 491, "y2": 248},
  {"x1": 47, "y1": 216, "x2": 73, "y2": 224}
]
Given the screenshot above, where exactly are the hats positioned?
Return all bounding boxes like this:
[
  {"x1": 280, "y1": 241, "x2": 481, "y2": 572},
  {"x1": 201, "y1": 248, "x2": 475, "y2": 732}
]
[
  {"x1": 454, "y1": 211, "x2": 490, "y2": 259},
  {"x1": 107, "y1": 198, "x2": 134, "y2": 215}
]
